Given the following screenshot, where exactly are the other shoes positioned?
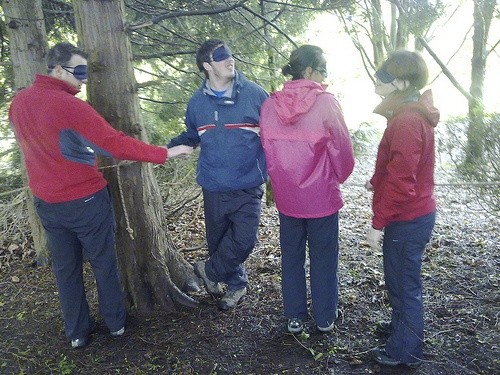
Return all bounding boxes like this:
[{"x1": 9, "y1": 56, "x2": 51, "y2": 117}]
[
  {"x1": 71, "y1": 335, "x2": 88, "y2": 348},
  {"x1": 287, "y1": 318, "x2": 303, "y2": 332},
  {"x1": 317, "y1": 321, "x2": 334, "y2": 332},
  {"x1": 220, "y1": 288, "x2": 246, "y2": 308},
  {"x1": 106, "y1": 321, "x2": 125, "y2": 338},
  {"x1": 374, "y1": 353, "x2": 422, "y2": 365},
  {"x1": 378, "y1": 320, "x2": 392, "y2": 334},
  {"x1": 193, "y1": 263, "x2": 223, "y2": 294}
]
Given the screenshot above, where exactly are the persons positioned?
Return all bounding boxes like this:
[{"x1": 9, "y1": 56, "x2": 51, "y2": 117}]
[
  {"x1": 259, "y1": 45, "x2": 355, "y2": 331},
  {"x1": 365, "y1": 50, "x2": 440, "y2": 368},
  {"x1": 7, "y1": 43, "x2": 192, "y2": 345},
  {"x1": 159, "y1": 39, "x2": 269, "y2": 310}
]
